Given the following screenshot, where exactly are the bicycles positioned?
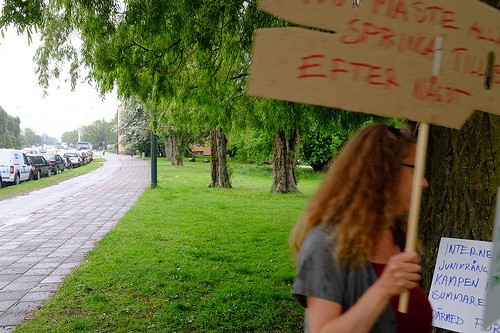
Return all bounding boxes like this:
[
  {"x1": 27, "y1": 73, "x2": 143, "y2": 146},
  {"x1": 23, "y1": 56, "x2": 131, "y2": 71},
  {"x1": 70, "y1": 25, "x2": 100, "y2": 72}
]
[{"x1": 102, "y1": 150, "x2": 105, "y2": 155}]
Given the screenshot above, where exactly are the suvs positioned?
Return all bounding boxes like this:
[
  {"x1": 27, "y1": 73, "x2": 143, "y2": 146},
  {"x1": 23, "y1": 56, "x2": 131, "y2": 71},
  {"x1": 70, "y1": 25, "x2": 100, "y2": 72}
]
[{"x1": 27, "y1": 155, "x2": 52, "y2": 180}]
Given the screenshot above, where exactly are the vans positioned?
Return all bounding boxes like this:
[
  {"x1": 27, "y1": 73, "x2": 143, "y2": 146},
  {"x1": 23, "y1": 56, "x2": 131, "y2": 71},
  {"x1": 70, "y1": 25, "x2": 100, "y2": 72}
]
[{"x1": 0, "y1": 149, "x2": 34, "y2": 185}]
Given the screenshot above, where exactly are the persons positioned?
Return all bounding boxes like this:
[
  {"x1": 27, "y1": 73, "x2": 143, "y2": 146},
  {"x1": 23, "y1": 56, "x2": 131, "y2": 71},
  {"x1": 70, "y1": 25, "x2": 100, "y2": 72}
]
[{"x1": 290, "y1": 122, "x2": 433, "y2": 333}]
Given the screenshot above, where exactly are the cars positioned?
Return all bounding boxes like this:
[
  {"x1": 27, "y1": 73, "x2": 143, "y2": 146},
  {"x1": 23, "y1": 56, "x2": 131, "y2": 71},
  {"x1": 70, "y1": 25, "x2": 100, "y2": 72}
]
[
  {"x1": 60, "y1": 142, "x2": 69, "y2": 150},
  {"x1": 62, "y1": 152, "x2": 82, "y2": 168},
  {"x1": 44, "y1": 155, "x2": 65, "y2": 175},
  {"x1": 61, "y1": 157, "x2": 73, "y2": 171},
  {"x1": 78, "y1": 146, "x2": 94, "y2": 165},
  {"x1": 0, "y1": 170, "x2": 2, "y2": 189},
  {"x1": 22, "y1": 144, "x2": 57, "y2": 155}
]
